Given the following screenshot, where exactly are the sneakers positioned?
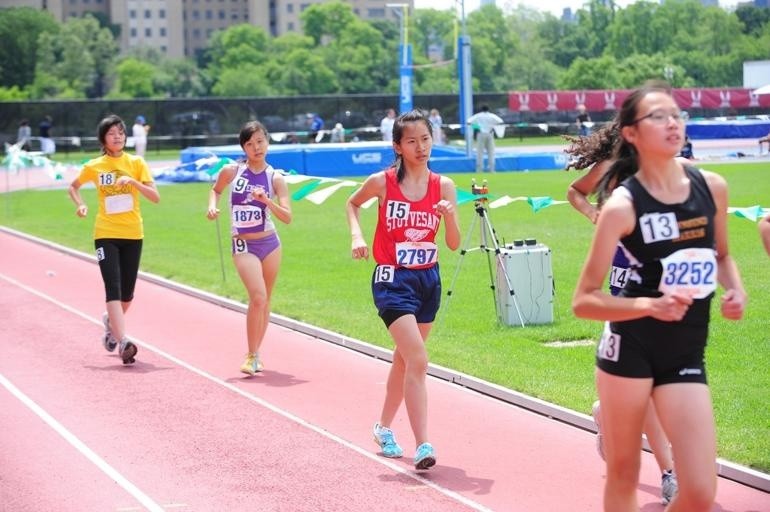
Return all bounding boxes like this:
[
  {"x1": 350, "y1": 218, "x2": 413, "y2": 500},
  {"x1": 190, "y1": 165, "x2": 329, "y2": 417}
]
[
  {"x1": 240, "y1": 353, "x2": 256, "y2": 376},
  {"x1": 256, "y1": 353, "x2": 263, "y2": 372},
  {"x1": 591, "y1": 401, "x2": 606, "y2": 462},
  {"x1": 414, "y1": 444, "x2": 438, "y2": 475},
  {"x1": 662, "y1": 470, "x2": 678, "y2": 507},
  {"x1": 372, "y1": 423, "x2": 404, "y2": 458},
  {"x1": 102, "y1": 313, "x2": 118, "y2": 352},
  {"x1": 119, "y1": 336, "x2": 138, "y2": 367}
]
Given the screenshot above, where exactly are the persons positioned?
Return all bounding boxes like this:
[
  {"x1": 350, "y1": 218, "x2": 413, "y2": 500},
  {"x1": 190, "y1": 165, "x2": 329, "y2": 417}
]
[
  {"x1": 67, "y1": 113, "x2": 160, "y2": 367},
  {"x1": 571, "y1": 87, "x2": 748, "y2": 512},
  {"x1": 132, "y1": 115, "x2": 151, "y2": 157},
  {"x1": 330, "y1": 122, "x2": 345, "y2": 143},
  {"x1": 346, "y1": 110, "x2": 461, "y2": 474},
  {"x1": 305, "y1": 113, "x2": 324, "y2": 143},
  {"x1": 680, "y1": 136, "x2": 695, "y2": 160},
  {"x1": 561, "y1": 108, "x2": 679, "y2": 506},
  {"x1": 428, "y1": 108, "x2": 445, "y2": 144},
  {"x1": 17, "y1": 119, "x2": 32, "y2": 151},
  {"x1": 576, "y1": 104, "x2": 595, "y2": 137},
  {"x1": 380, "y1": 108, "x2": 396, "y2": 141},
  {"x1": 39, "y1": 115, "x2": 56, "y2": 158},
  {"x1": 466, "y1": 105, "x2": 505, "y2": 173},
  {"x1": 206, "y1": 120, "x2": 292, "y2": 378}
]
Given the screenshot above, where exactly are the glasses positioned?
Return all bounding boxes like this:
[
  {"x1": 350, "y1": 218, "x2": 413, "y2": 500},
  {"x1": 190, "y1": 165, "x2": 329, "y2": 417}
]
[{"x1": 624, "y1": 110, "x2": 689, "y2": 124}]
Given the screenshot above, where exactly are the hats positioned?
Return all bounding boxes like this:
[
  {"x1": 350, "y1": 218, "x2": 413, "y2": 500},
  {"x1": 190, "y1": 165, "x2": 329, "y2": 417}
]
[{"x1": 136, "y1": 116, "x2": 145, "y2": 122}]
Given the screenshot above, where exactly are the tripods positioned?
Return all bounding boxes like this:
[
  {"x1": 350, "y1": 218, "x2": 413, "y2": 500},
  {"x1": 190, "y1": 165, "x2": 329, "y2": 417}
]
[{"x1": 440, "y1": 186, "x2": 524, "y2": 327}]
